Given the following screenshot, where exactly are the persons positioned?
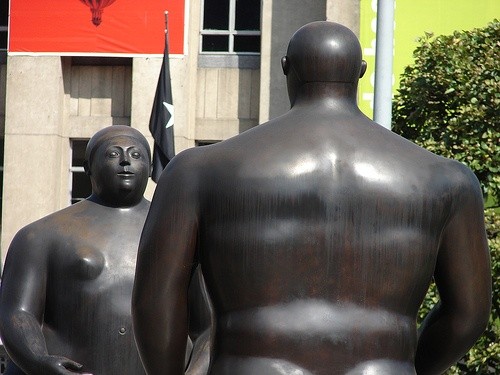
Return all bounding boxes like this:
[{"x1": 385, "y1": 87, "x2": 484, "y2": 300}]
[
  {"x1": 0, "y1": 125, "x2": 211, "y2": 375},
  {"x1": 130, "y1": 21, "x2": 493, "y2": 375}
]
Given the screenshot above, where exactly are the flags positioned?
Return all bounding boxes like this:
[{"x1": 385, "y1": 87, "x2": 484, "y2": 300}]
[{"x1": 148, "y1": 32, "x2": 175, "y2": 184}]
